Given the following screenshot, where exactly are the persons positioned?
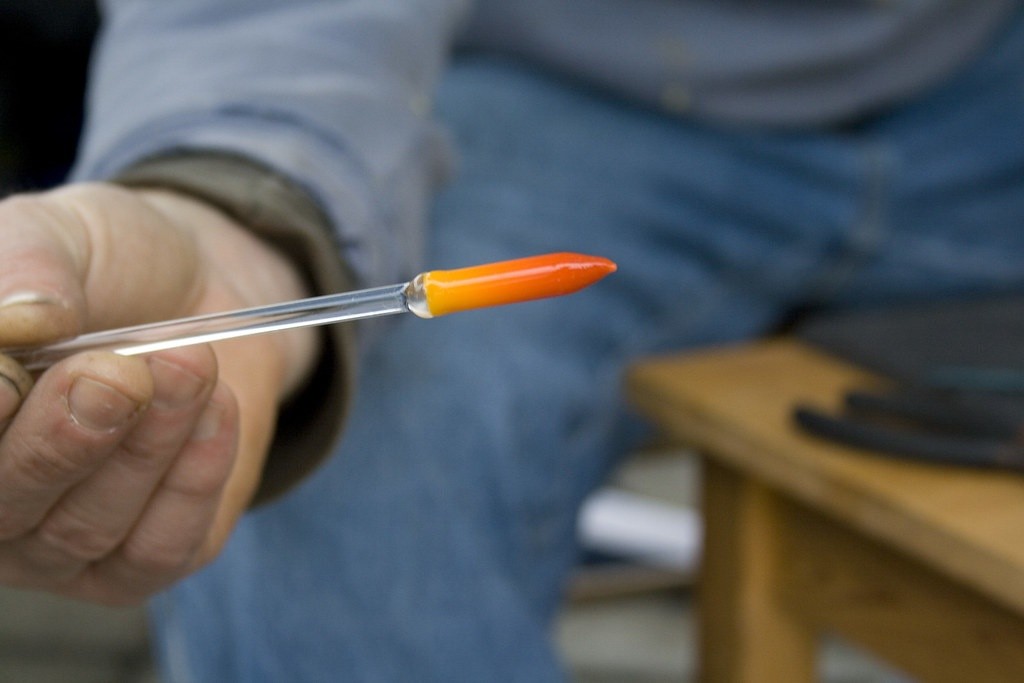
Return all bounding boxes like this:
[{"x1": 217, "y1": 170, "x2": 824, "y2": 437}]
[{"x1": 0, "y1": 0, "x2": 1024, "y2": 683}]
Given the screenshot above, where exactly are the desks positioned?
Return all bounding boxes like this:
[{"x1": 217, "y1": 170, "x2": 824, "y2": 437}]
[{"x1": 624, "y1": 338, "x2": 1024, "y2": 683}]
